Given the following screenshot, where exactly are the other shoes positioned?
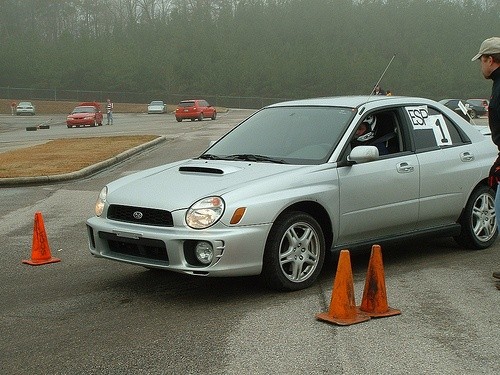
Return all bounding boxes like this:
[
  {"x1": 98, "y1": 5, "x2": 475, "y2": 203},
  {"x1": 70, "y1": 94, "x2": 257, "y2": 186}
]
[
  {"x1": 492, "y1": 271, "x2": 500, "y2": 279},
  {"x1": 495, "y1": 282, "x2": 500, "y2": 291}
]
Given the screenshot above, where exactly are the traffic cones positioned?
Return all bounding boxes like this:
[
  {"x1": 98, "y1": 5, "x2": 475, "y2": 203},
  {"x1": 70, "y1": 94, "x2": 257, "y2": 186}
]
[
  {"x1": 356, "y1": 244, "x2": 402, "y2": 319},
  {"x1": 313, "y1": 249, "x2": 371, "y2": 326},
  {"x1": 21, "y1": 212, "x2": 62, "y2": 266}
]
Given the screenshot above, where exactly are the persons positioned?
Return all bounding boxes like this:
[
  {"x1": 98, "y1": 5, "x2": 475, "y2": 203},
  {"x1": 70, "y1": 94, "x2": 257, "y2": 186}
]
[
  {"x1": 386, "y1": 90, "x2": 391, "y2": 95},
  {"x1": 472, "y1": 37, "x2": 500, "y2": 289},
  {"x1": 350, "y1": 113, "x2": 390, "y2": 158},
  {"x1": 106, "y1": 99, "x2": 114, "y2": 125},
  {"x1": 372, "y1": 84, "x2": 386, "y2": 95},
  {"x1": 11, "y1": 102, "x2": 17, "y2": 115}
]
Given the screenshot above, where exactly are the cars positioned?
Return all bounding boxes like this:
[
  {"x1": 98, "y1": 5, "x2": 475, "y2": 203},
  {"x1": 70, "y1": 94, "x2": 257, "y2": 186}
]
[
  {"x1": 66, "y1": 102, "x2": 103, "y2": 128},
  {"x1": 174, "y1": 99, "x2": 217, "y2": 123},
  {"x1": 85, "y1": 96, "x2": 498, "y2": 293},
  {"x1": 466, "y1": 99, "x2": 490, "y2": 111},
  {"x1": 437, "y1": 98, "x2": 488, "y2": 120},
  {"x1": 16, "y1": 101, "x2": 37, "y2": 116},
  {"x1": 147, "y1": 100, "x2": 167, "y2": 114}
]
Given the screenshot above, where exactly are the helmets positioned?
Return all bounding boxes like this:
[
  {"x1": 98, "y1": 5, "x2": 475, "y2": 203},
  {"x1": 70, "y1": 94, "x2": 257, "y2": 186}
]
[{"x1": 356, "y1": 115, "x2": 379, "y2": 143}]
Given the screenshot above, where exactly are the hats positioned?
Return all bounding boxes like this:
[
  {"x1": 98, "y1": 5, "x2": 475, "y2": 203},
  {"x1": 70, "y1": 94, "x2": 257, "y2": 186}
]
[{"x1": 472, "y1": 36, "x2": 500, "y2": 62}]
